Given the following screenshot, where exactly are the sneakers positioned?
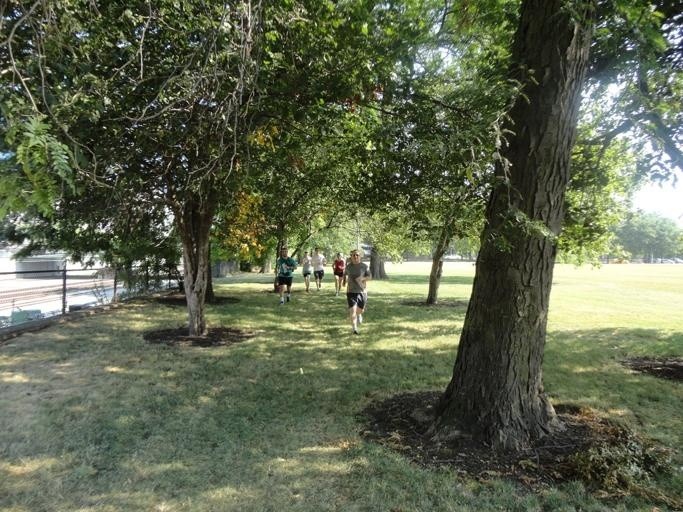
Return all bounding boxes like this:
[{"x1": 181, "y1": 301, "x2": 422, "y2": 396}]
[
  {"x1": 279, "y1": 294, "x2": 290, "y2": 305},
  {"x1": 352, "y1": 315, "x2": 362, "y2": 333}
]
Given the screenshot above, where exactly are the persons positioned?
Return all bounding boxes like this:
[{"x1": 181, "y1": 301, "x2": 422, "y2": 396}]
[
  {"x1": 345, "y1": 251, "x2": 353, "y2": 263},
  {"x1": 275, "y1": 248, "x2": 297, "y2": 305},
  {"x1": 343, "y1": 249, "x2": 372, "y2": 334},
  {"x1": 300, "y1": 252, "x2": 312, "y2": 293},
  {"x1": 311, "y1": 248, "x2": 327, "y2": 291},
  {"x1": 332, "y1": 252, "x2": 346, "y2": 296}
]
